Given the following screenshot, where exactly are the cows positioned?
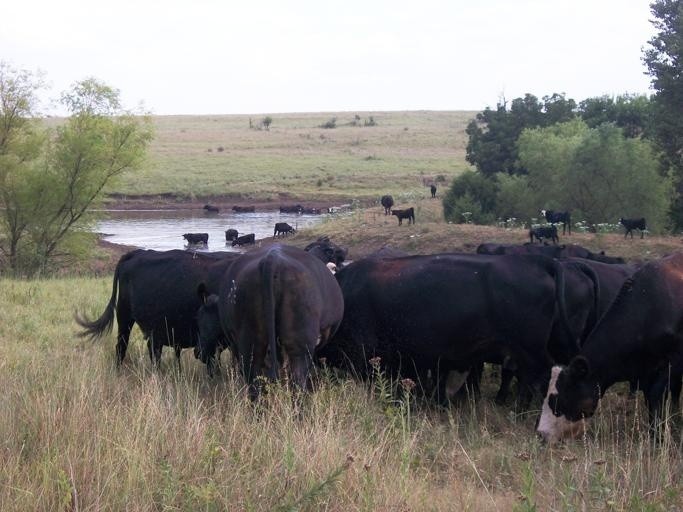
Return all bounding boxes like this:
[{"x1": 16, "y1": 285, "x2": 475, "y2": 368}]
[
  {"x1": 279, "y1": 202, "x2": 322, "y2": 216},
  {"x1": 304, "y1": 234, "x2": 348, "y2": 276},
  {"x1": 528, "y1": 225, "x2": 561, "y2": 244},
  {"x1": 431, "y1": 185, "x2": 436, "y2": 197},
  {"x1": 316, "y1": 249, "x2": 579, "y2": 414},
  {"x1": 495, "y1": 251, "x2": 649, "y2": 406},
  {"x1": 380, "y1": 194, "x2": 416, "y2": 226},
  {"x1": 617, "y1": 216, "x2": 646, "y2": 239},
  {"x1": 74, "y1": 250, "x2": 241, "y2": 373},
  {"x1": 533, "y1": 251, "x2": 682, "y2": 448},
  {"x1": 193, "y1": 244, "x2": 345, "y2": 419},
  {"x1": 203, "y1": 202, "x2": 257, "y2": 214},
  {"x1": 474, "y1": 235, "x2": 625, "y2": 264},
  {"x1": 538, "y1": 208, "x2": 575, "y2": 235}
]
